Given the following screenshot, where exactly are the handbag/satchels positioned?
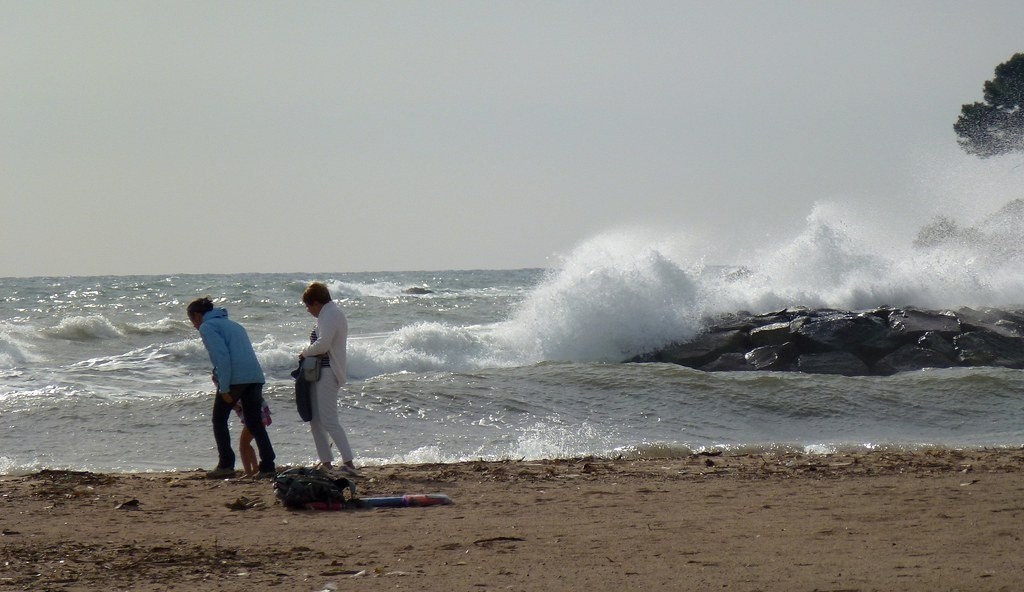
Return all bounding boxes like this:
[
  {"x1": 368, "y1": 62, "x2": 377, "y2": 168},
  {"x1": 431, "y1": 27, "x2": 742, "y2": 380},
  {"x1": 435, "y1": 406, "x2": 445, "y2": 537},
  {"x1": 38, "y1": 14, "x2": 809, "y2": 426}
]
[{"x1": 302, "y1": 356, "x2": 320, "y2": 382}]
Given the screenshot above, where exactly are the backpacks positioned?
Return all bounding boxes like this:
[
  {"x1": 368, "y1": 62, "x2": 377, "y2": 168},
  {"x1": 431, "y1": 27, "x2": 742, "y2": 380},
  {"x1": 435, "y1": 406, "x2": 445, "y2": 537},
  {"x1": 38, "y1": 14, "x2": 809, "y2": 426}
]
[{"x1": 273, "y1": 466, "x2": 356, "y2": 513}]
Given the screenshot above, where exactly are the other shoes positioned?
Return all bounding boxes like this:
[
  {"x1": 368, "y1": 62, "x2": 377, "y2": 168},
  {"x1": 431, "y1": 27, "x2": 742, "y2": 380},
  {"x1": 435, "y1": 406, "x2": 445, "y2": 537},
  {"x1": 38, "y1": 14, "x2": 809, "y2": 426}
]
[
  {"x1": 252, "y1": 470, "x2": 276, "y2": 480},
  {"x1": 207, "y1": 467, "x2": 234, "y2": 479}
]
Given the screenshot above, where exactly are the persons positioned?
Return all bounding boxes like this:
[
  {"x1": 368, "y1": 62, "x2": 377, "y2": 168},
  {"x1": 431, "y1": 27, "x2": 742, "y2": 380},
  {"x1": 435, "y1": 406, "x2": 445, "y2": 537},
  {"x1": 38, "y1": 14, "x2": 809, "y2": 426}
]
[
  {"x1": 295, "y1": 281, "x2": 355, "y2": 470},
  {"x1": 212, "y1": 375, "x2": 272, "y2": 480},
  {"x1": 187, "y1": 294, "x2": 279, "y2": 480}
]
